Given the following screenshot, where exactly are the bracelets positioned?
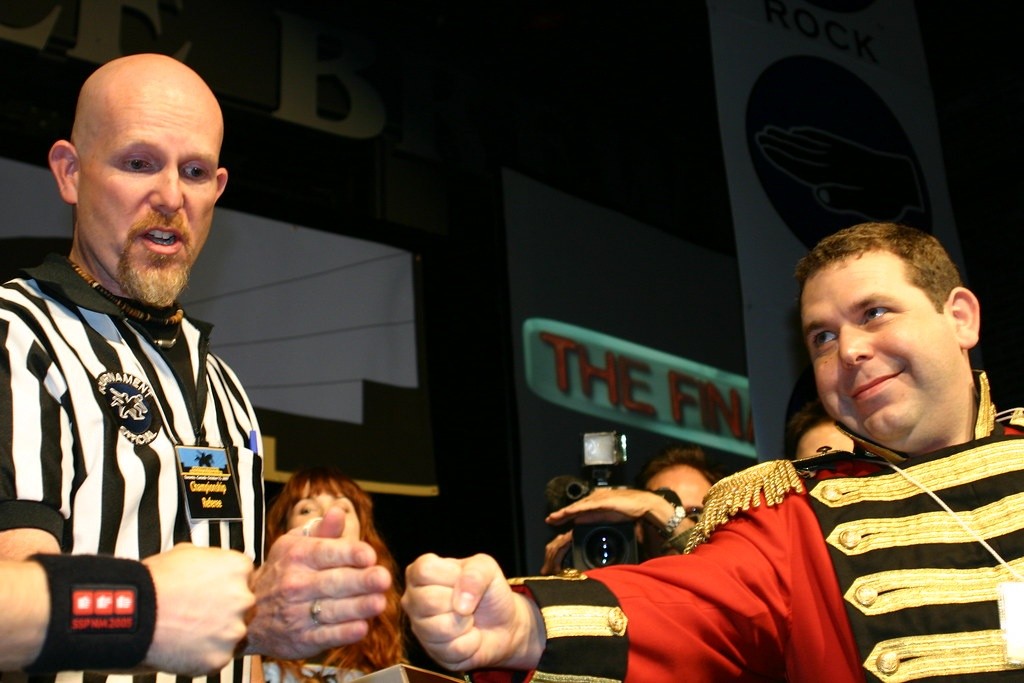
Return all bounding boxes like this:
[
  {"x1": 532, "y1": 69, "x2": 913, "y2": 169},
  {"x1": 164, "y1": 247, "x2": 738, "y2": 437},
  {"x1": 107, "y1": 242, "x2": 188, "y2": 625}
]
[{"x1": 29, "y1": 552, "x2": 157, "y2": 672}]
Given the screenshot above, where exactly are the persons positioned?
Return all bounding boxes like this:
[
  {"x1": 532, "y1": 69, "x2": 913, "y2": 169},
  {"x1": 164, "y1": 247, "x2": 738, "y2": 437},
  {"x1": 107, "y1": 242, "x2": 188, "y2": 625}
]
[
  {"x1": 262, "y1": 467, "x2": 408, "y2": 683},
  {"x1": 400, "y1": 221, "x2": 1024, "y2": 683},
  {"x1": 541, "y1": 449, "x2": 719, "y2": 575},
  {"x1": 0, "y1": 52, "x2": 392, "y2": 683},
  {"x1": 0, "y1": 542, "x2": 258, "y2": 677},
  {"x1": 788, "y1": 398, "x2": 860, "y2": 462}
]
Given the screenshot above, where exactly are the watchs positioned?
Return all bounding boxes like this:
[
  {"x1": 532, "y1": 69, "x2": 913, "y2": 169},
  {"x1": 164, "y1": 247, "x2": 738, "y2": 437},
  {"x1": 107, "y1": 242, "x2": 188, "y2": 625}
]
[{"x1": 665, "y1": 505, "x2": 687, "y2": 535}]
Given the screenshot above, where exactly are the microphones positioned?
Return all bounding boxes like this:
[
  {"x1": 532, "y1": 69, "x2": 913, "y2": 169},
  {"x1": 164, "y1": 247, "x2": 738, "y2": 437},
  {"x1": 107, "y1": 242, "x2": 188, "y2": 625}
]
[{"x1": 545, "y1": 475, "x2": 589, "y2": 500}]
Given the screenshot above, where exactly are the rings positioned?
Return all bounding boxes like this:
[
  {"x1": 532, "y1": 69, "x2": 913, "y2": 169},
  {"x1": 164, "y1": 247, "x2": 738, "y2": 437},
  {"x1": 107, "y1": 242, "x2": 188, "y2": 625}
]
[{"x1": 311, "y1": 598, "x2": 321, "y2": 625}]
